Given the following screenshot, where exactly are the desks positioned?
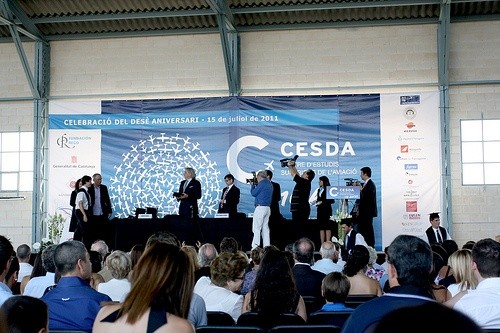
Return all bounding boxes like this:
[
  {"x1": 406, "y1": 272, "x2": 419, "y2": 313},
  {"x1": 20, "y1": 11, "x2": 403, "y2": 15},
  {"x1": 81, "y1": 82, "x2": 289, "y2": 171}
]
[{"x1": 81, "y1": 218, "x2": 339, "y2": 264}]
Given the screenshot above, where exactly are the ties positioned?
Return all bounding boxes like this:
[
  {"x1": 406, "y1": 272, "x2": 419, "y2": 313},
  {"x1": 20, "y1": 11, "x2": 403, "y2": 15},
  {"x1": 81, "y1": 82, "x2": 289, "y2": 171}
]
[
  {"x1": 436, "y1": 230, "x2": 442, "y2": 243},
  {"x1": 347, "y1": 235, "x2": 349, "y2": 244},
  {"x1": 222, "y1": 188, "x2": 228, "y2": 200},
  {"x1": 183, "y1": 180, "x2": 188, "y2": 193}
]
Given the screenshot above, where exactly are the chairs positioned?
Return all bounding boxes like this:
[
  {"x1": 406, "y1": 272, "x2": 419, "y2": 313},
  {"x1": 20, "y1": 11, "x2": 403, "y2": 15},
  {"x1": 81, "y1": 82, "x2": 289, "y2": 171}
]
[{"x1": 194, "y1": 294, "x2": 378, "y2": 333}]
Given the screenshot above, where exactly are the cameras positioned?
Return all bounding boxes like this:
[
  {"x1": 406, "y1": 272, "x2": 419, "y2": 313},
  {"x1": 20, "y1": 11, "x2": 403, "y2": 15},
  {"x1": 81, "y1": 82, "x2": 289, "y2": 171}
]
[
  {"x1": 280, "y1": 155, "x2": 298, "y2": 168},
  {"x1": 246, "y1": 172, "x2": 257, "y2": 188}
]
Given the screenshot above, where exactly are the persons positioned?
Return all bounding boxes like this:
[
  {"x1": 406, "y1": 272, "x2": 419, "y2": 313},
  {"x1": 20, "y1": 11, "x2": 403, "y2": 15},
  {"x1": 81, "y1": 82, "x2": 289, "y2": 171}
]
[
  {"x1": 0, "y1": 228, "x2": 419, "y2": 333},
  {"x1": 288, "y1": 160, "x2": 315, "y2": 246},
  {"x1": 217, "y1": 173, "x2": 242, "y2": 217},
  {"x1": 350, "y1": 167, "x2": 378, "y2": 250},
  {"x1": 419, "y1": 238, "x2": 500, "y2": 333},
  {"x1": 91, "y1": 240, "x2": 208, "y2": 333},
  {"x1": 65, "y1": 173, "x2": 112, "y2": 249},
  {"x1": 315, "y1": 176, "x2": 336, "y2": 247},
  {"x1": 339, "y1": 219, "x2": 369, "y2": 261},
  {"x1": 248, "y1": 169, "x2": 282, "y2": 261},
  {"x1": 419, "y1": 212, "x2": 455, "y2": 249},
  {"x1": 176, "y1": 168, "x2": 205, "y2": 218},
  {"x1": 346, "y1": 232, "x2": 471, "y2": 333}
]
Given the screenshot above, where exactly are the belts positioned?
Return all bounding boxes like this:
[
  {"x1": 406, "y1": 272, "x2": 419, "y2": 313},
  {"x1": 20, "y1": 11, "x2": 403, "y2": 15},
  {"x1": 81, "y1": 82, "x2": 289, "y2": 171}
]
[{"x1": 257, "y1": 205, "x2": 268, "y2": 206}]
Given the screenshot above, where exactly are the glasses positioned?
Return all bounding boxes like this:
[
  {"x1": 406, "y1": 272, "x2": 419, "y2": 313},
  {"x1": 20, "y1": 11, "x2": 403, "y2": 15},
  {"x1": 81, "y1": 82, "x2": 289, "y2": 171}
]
[
  {"x1": 233, "y1": 273, "x2": 245, "y2": 280},
  {"x1": 224, "y1": 179, "x2": 230, "y2": 182}
]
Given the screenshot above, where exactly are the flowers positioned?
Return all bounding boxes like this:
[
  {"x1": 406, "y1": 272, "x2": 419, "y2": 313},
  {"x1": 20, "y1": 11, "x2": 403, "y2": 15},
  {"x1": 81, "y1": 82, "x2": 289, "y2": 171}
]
[
  {"x1": 330, "y1": 209, "x2": 352, "y2": 241},
  {"x1": 44, "y1": 211, "x2": 65, "y2": 246}
]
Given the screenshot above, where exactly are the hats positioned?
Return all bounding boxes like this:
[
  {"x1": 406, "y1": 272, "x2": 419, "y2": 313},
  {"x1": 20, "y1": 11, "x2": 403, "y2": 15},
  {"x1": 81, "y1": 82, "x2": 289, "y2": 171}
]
[{"x1": 428, "y1": 212, "x2": 440, "y2": 222}]
